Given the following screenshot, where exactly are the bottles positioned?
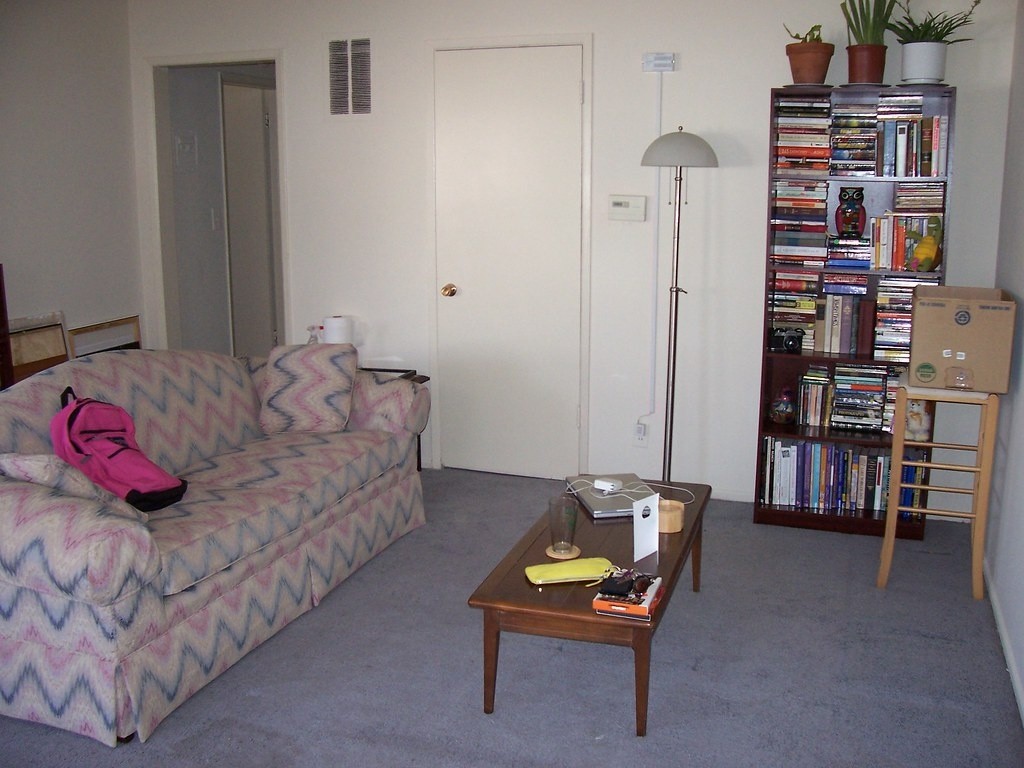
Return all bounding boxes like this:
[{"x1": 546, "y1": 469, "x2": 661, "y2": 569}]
[
  {"x1": 659, "y1": 500, "x2": 684, "y2": 533},
  {"x1": 835, "y1": 186, "x2": 866, "y2": 238},
  {"x1": 548, "y1": 496, "x2": 579, "y2": 553},
  {"x1": 769, "y1": 396, "x2": 793, "y2": 423}
]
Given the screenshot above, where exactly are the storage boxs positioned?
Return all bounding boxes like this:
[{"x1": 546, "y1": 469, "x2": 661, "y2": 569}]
[{"x1": 907, "y1": 282, "x2": 1018, "y2": 395}]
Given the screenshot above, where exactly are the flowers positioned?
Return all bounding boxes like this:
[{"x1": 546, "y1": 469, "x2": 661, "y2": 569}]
[{"x1": 884, "y1": 0, "x2": 981, "y2": 45}]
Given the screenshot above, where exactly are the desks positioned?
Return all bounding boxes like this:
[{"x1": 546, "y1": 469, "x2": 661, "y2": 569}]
[{"x1": 874, "y1": 375, "x2": 1000, "y2": 601}]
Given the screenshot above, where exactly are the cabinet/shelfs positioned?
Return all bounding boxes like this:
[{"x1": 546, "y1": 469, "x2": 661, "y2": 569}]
[{"x1": 752, "y1": 86, "x2": 959, "y2": 540}]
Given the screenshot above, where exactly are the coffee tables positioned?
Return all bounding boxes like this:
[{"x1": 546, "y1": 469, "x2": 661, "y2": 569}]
[{"x1": 468, "y1": 479, "x2": 712, "y2": 735}]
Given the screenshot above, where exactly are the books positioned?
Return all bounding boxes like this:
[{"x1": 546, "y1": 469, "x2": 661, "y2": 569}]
[
  {"x1": 883, "y1": 182, "x2": 945, "y2": 271},
  {"x1": 760, "y1": 436, "x2": 927, "y2": 517},
  {"x1": 829, "y1": 102, "x2": 884, "y2": 178},
  {"x1": 874, "y1": 90, "x2": 948, "y2": 178},
  {"x1": 776, "y1": 97, "x2": 831, "y2": 176},
  {"x1": 796, "y1": 361, "x2": 899, "y2": 433},
  {"x1": 826, "y1": 215, "x2": 892, "y2": 269},
  {"x1": 813, "y1": 272, "x2": 875, "y2": 357},
  {"x1": 772, "y1": 178, "x2": 831, "y2": 268},
  {"x1": 874, "y1": 274, "x2": 940, "y2": 363},
  {"x1": 771, "y1": 269, "x2": 821, "y2": 350}
]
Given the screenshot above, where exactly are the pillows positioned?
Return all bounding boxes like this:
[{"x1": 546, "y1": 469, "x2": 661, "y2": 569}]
[
  {"x1": 1, "y1": 454, "x2": 149, "y2": 525},
  {"x1": 259, "y1": 343, "x2": 358, "y2": 437}
]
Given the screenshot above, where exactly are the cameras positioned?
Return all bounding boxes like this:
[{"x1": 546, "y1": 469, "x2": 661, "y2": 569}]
[{"x1": 768, "y1": 327, "x2": 806, "y2": 352}]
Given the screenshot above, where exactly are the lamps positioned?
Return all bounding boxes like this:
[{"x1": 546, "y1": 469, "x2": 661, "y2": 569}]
[{"x1": 640, "y1": 125, "x2": 720, "y2": 479}]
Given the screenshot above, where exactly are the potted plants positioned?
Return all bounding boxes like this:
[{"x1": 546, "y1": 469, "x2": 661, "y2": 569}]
[
  {"x1": 840, "y1": 0, "x2": 896, "y2": 84},
  {"x1": 783, "y1": 23, "x2": 835, "y2": 85}
]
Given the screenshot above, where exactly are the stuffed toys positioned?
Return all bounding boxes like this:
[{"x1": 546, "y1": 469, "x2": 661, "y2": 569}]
[{"x1": 904, "y1": 399, "x2": 933, "y2": 442}]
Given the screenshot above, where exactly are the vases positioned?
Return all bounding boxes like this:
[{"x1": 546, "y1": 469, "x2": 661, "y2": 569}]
[{"x1": 901, "y1": 42, "x2": 947, "y2": 85}]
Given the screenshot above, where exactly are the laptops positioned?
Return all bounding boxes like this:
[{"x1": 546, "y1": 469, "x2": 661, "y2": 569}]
[{"x1": 564, "y1": 472, "x2": 662, "y2": 519}]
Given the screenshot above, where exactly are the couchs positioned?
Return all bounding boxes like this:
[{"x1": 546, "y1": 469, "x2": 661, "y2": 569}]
[{"x1": 0, "y1": 349, "x2": 432, "y2": 747}]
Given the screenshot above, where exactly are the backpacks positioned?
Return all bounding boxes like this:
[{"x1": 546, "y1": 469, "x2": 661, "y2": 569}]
[{"x1": 51, "y1": 385, "x2": 187, "y2": 512}]
